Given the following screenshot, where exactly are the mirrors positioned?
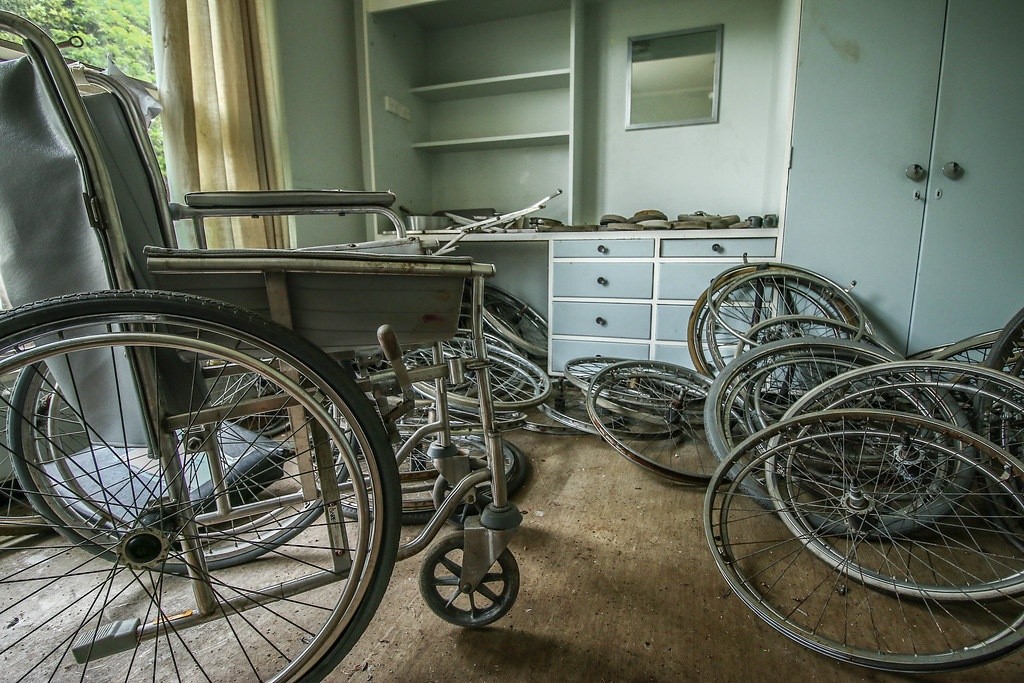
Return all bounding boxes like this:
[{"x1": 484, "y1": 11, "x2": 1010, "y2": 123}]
[{"x1": 623, "y1": 23, "x2": 724, "y2": 131}]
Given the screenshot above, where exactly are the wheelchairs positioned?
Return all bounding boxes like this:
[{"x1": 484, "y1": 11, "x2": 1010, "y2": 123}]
[{"x1": 0, "y1": 10, "x2": 522, "y2": 683}]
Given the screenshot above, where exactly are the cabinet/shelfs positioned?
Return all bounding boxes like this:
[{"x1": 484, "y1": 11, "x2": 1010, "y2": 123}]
[
  {"x1": 546, "y1": 227, "x2": 778, "y2": 397},
  {"x1": 353, "y1": 0, "x2": 587, "y2": 247},
  {"x1": 765, "y1": 0, "x2": 1024, "y2": 395}
]
